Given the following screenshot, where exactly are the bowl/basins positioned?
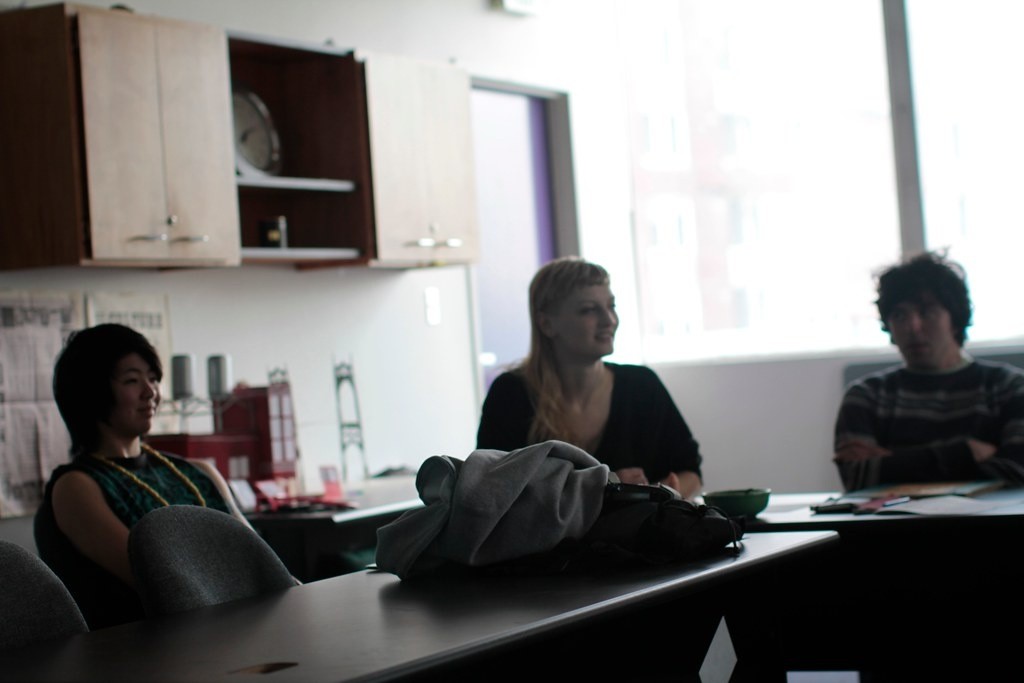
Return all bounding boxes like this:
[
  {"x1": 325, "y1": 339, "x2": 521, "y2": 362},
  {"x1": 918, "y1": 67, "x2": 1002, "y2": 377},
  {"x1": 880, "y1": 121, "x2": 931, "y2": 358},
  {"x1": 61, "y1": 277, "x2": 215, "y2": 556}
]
[{"x1": 702, "y1": 489, "x2": 771, "y2": 520}]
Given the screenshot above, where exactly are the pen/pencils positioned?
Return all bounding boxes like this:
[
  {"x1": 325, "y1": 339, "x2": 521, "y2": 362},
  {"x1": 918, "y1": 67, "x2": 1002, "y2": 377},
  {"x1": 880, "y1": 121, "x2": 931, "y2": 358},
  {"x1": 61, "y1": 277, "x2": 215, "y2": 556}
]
[{"x1": 883, "y1": 496, "x2": 910, "y2": 507}]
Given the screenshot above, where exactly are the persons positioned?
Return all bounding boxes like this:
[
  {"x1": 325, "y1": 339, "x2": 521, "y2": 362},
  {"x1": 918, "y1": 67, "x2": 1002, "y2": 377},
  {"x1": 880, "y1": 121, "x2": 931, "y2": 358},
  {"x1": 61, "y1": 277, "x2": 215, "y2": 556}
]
[
  {"x1": 476, "y1": 258, "x2": 705, "y2": 502},
  {"x1": 832, "y1": 254, "x2": 1024, "y2": 683},
  {"x1": 33, "y1": 323, "x2": 302, "y2": 632}
]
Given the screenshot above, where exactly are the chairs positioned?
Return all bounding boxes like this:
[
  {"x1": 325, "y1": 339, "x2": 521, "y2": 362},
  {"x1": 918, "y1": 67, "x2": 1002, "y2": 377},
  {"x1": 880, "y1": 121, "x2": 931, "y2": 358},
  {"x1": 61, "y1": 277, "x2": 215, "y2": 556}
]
[
  {"x1": 130, "y1": 500, "x2": 293, "y2": 618},
  {"x1": 1, "y1": 541, "x2": 83, "y2": 660}
]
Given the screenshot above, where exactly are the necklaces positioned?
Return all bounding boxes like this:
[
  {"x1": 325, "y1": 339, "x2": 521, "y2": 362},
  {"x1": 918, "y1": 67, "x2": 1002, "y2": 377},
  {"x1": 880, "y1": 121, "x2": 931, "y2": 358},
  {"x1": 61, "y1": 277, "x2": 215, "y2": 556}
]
[{"x1": 96, "y1": 443, "x2": 206, "y2": 505}]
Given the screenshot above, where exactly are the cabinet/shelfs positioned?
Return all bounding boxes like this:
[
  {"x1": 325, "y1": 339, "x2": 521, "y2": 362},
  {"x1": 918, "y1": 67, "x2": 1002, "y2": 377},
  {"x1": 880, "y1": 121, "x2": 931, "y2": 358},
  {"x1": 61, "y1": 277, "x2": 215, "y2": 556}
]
[{"x1": 0, "y1": 2, "x2": 475, "y2": 278}]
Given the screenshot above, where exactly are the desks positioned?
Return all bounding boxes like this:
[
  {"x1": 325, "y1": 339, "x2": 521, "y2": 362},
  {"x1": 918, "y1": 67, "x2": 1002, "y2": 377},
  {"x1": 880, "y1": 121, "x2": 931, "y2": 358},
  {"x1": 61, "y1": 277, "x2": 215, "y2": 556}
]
[{"x1": 0, "y1": 488, "x2": 1023, "y2": 683}]
